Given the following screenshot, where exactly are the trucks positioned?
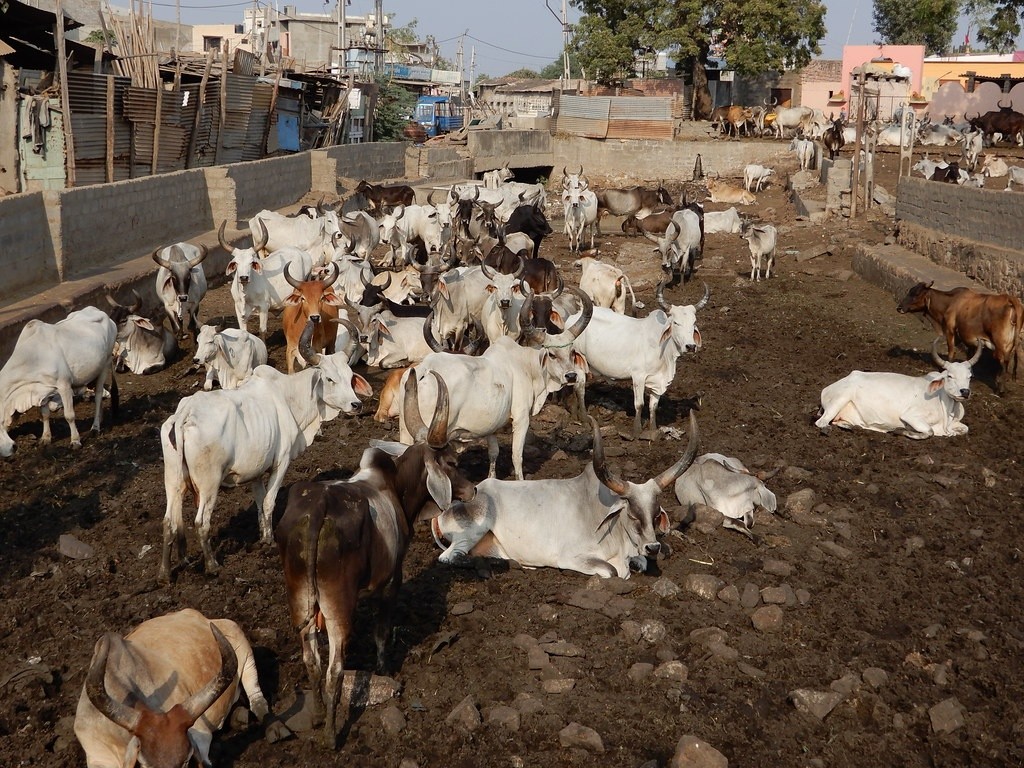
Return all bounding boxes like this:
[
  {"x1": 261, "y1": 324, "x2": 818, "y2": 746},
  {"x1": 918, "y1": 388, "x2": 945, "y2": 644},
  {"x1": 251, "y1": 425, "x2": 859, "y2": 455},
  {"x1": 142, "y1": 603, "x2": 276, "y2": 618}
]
[{"x1": 403, "y1": 96, "x2": 463, "y2": 144}]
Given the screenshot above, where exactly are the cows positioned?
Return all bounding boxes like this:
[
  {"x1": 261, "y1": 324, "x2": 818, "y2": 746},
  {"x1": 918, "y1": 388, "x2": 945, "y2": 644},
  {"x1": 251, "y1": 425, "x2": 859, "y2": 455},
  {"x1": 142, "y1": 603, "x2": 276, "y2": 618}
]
[
  {"x1": 714, "y1": 98, "x2": 1024, "y2": 197},
  {"x1": 0, "y1": 162, "x2": 1024, "y2": 768}
]
